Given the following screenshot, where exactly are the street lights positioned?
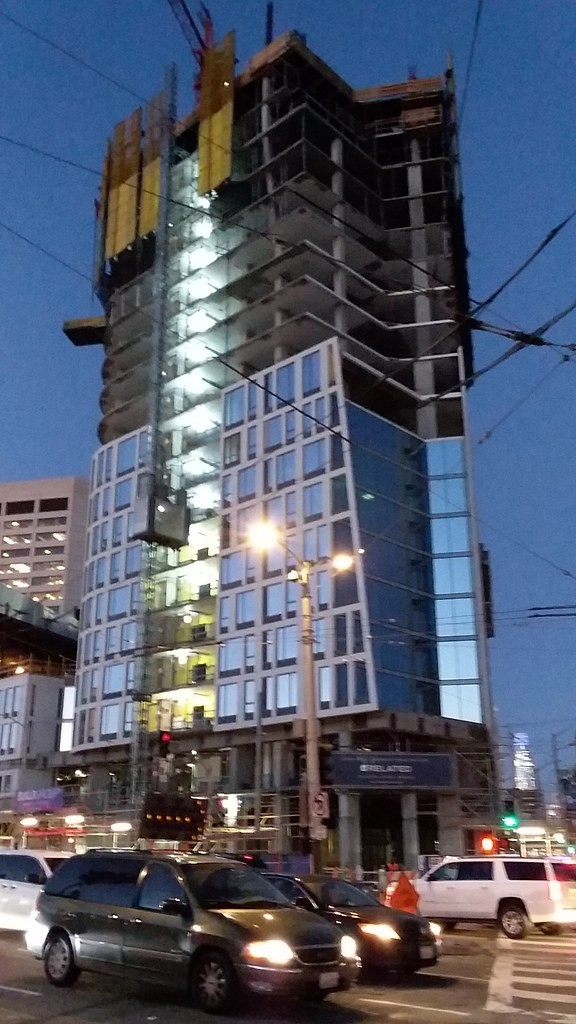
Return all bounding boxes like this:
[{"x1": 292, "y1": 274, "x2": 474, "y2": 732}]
[{"x1": 244, "y1": 517, "x2": 354, "y2": 870}]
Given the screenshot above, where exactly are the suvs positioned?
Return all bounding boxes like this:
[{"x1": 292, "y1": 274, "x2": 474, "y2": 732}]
[
  {"x1": 401, "y1": 855, "x2": 576, "y2": 941},
  {"x1": 26, "y1": 846, "x2": 363, "y2": 1016}
]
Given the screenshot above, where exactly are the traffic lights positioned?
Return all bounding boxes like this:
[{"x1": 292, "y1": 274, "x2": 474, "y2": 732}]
[
  {"x1": 503, "y1": 800, "x2": 517, "y2": 828},
  {"x1": 159, "y1": 730, "x2": 171, "y2": 759}
]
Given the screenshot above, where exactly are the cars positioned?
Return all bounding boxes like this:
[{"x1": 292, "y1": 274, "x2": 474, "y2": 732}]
[
  {"x1": 259, "y1": 869, "x2": 439, "y2": 986},
  {"x1": 0, "y1": 848, "x2": 82, "y2": 932}
]
